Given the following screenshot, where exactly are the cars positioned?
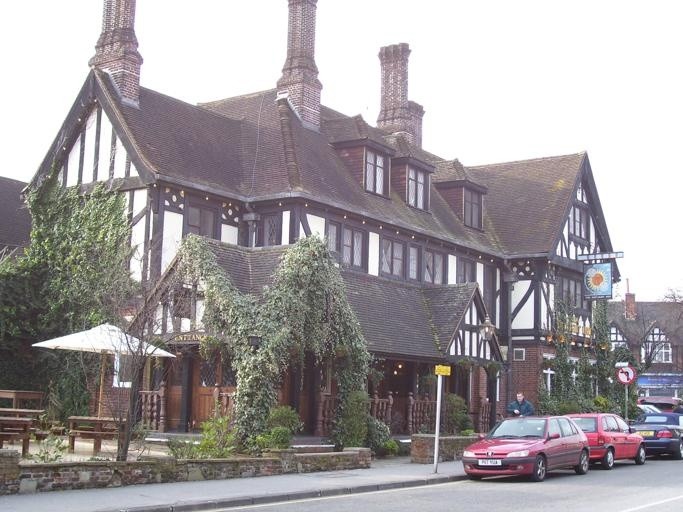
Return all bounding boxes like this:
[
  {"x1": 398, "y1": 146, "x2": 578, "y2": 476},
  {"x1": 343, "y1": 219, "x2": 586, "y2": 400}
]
[
  {"x1": 461, "y1": 413, "x2": 683, "y2": 481},
  {"x1": 632, "y1": 396, "x2": 683, "y2": 413}
]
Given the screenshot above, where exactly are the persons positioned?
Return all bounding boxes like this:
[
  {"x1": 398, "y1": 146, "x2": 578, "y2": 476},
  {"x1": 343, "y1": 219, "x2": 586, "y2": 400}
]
[
  {"x1": 506, "y1": 391, "x2": 535, "y2": 417},
  {"x1": 672, "y1": 403, "x2": 683, "y2": 414}
]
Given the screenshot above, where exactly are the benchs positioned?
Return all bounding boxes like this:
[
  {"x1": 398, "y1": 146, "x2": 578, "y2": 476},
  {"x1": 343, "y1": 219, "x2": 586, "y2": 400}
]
[
  {"x1": 0, "y1": 407, "x2": 44, "y2": 418},
  {"x1": 0, "y1": 427, "x2": 37, "y2": 440},
  {"x1": 67, "y1": 425, "x2": 139, "y2": 442}
]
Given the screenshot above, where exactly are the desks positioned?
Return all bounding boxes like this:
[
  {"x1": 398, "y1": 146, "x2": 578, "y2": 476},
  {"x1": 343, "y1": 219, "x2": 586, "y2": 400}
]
[
  {"x1": 67, "y1": 415, "x2": 126, "y2": 454},
  {"x1": 0, "y1": 389, "x2": 45, "y2": 420},
  {"x1": 0, "y1": 416, "x2": 38, "y2": 459}
]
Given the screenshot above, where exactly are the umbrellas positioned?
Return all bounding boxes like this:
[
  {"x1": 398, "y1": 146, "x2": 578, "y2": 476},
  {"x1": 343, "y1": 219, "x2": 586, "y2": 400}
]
[{"x1": 31, "y1": 321, "x2": 178, "y2": 419}]
